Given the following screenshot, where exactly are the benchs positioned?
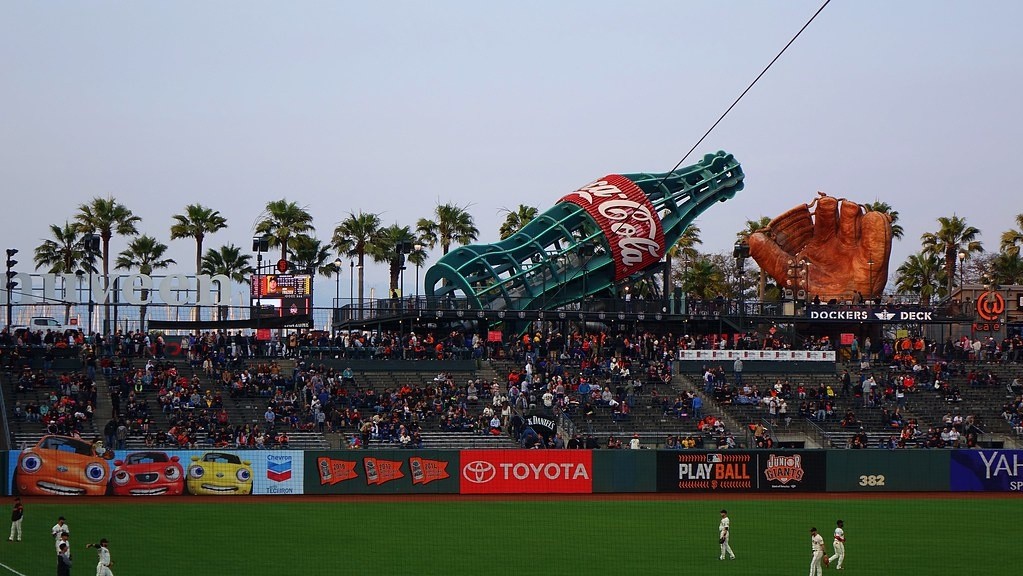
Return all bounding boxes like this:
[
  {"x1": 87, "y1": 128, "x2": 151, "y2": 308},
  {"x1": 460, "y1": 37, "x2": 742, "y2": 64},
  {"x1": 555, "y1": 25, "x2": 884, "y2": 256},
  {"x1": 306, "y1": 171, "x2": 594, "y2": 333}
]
[{"x1": 0, "y1": 347, "x2": 1023, "y2": 449}]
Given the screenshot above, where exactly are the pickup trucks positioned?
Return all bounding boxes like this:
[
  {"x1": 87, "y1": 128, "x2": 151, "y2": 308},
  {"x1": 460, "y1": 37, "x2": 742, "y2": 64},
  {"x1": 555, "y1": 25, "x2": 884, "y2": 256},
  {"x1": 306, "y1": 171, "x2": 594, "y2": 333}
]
[{"x1": 5, "y1": 317, "x2": 79, "y2": 342}]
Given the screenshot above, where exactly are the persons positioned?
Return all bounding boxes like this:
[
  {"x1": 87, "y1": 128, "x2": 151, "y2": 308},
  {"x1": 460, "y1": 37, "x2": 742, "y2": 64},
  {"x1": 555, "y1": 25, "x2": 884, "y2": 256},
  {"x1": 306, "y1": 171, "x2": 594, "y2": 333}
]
[
  {"x1": 1, "y1": 289, "x2": 1023, "y2": 450},
  {"x1": 717, "y1": 509, "x2": 736, "y2": 561},
  {"x1": 51, "y1": 516, "x2": 72, "y2": 576},
  {"x1": 85, "y1": 538, "x2": 113, "y2": 576},
  {"x1": 826, "y1": 520, "x2": 847, "y2": 570},
  {"x1": 6, "y1": 497, "x2": 24, "y2": 542},
  {"x1": 809, "y1": 527, "x2": 826, "y2": 576}
]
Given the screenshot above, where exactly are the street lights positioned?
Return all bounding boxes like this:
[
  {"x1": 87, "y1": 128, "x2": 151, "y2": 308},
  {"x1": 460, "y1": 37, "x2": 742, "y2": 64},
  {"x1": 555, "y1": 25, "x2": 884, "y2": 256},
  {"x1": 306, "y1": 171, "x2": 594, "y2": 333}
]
[
  {"x1": 85, "y1": 236, "x2": 100, "y2": 337},
  {"x1": 958, "y1": 251, "x2": 965, "y2": 304},
  {"x1": 395, "y1": 241, "x2": 411, "y2": 335},
  {"x1": 683, "y1": 254, "x2": 691, "y2": 314},
  {"x1": 787, "y1": 259, "x2": 807, "y2": 317},
  {"x1": 335, "y1": 258, "x2": 342, "y2": 322},
  {"x1": 577, "y1": 242, "x2": 593, "y2": 336},
  {"x1": 867, "y1": 253, "x2": 874, "y2": 305},
  {"x1": 253, "y1": 236, "x2": 268, "y2": 307},
  {"x1": 414, "y1": 241, "x2": 422, "y2": 309},
  {"x1": 733, "y1": 245, "x2": 749, "y2": 315},
  {"x1": 805, "y1": 258, "x2": 812, "y2": 318},
  {"x1": 75, "y1": 270, "x2": 86, "y2": 301}
]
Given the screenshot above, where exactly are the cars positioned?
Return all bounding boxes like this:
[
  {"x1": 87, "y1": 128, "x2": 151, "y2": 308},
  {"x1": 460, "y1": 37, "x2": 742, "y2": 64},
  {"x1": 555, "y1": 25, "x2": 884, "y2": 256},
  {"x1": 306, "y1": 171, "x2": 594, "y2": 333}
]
[
  {"x1": 109, "y1": 452, "x2": 184, "y2": 496},
  {"x1": 12, "y1": 434, "x2": 115, "y2": 497},
  {"x1": 186, "y1": 452, "x2": 253, "y2": 496}
]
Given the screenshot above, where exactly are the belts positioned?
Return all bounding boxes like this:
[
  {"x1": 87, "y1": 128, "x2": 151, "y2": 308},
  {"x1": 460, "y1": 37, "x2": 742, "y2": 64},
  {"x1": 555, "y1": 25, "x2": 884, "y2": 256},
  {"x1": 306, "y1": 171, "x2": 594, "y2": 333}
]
[{"x1": 812, "y1": 550, "x2": 817, "y2": 551}]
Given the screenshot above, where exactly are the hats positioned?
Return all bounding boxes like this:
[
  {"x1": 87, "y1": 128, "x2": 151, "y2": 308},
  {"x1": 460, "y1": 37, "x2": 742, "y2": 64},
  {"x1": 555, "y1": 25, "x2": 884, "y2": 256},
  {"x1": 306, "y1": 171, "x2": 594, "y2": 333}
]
[
  {"x1": 270, "y1": 277, "x2": 277, "y2": 283},
  {"x1": 100, "y1": 539, "x2": 110, "y2": 543},
  {"x1": 779, "y1": 399, "x2": 784, "y2": 402},
  {"x1": 268, "y1": 407, "x2": 271, "y2": 409},
  {"x1": 14, "y1": 497, "x2": 22, "y2": 501},
  {"x1": 765, "y1": 435, "x2": 769, "y2": 438},
  {"x1": 720, "y1": 509, "x2": 727, "y2": 513},
  {"x1": 634, "y1": 435, "x2": 639, "y2": 438},
  {"x1": 206, "y1": 390, "x2": 211, "y2": 394},
  {"x1": 837, "y1": 520, "x2": 844, "y2": 524},
  {"x1": 59, "y1": 517, "x2": 66, "y2": 520},
  {"x1": 61, "y1": 532, "x2": 69, "y2": 536},
  {"x1": 810, "y1": 527, "x2": 817, "y2": 531}
]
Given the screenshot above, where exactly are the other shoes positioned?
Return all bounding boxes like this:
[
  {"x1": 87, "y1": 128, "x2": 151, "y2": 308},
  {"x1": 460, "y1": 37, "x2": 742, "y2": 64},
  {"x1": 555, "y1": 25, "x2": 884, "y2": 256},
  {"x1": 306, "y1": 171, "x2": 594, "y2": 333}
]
[
  {"x1": 836, "y1": 567, "x2": 845, "y2": 570},
  {"x1": 773, "y1": 424, "x2": 778, "y2": 426},
  {"x1": 587, "y1": 420, "x2": 592, "y2": 423},
  {"x1": 1010, "y1": 361, "x2": 1015, "y2": 365},
  {"x1": 863, "y1": 405, "x2": 866, "y2": 407}
]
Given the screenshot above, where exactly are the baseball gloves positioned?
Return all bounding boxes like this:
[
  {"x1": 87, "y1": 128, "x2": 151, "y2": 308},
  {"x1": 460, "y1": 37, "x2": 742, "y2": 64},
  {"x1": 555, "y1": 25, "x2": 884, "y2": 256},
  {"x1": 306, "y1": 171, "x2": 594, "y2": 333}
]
[{"x1": 748, "y1": 194, "x2": 893, "y2": 304}]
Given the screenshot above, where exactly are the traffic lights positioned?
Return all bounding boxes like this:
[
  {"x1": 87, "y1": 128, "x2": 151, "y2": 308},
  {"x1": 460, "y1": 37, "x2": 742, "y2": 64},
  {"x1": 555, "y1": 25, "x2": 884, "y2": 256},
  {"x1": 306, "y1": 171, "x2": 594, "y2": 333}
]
[
  {"x1": 70, "y1": 319, "x2": 77, "y2": 325},
  {"x1": 6, "y1": 249, "x2": 18, "y2": 289},
  {"x1": 277, "y1": 259, "x2": 288, "y2": 272}
]
